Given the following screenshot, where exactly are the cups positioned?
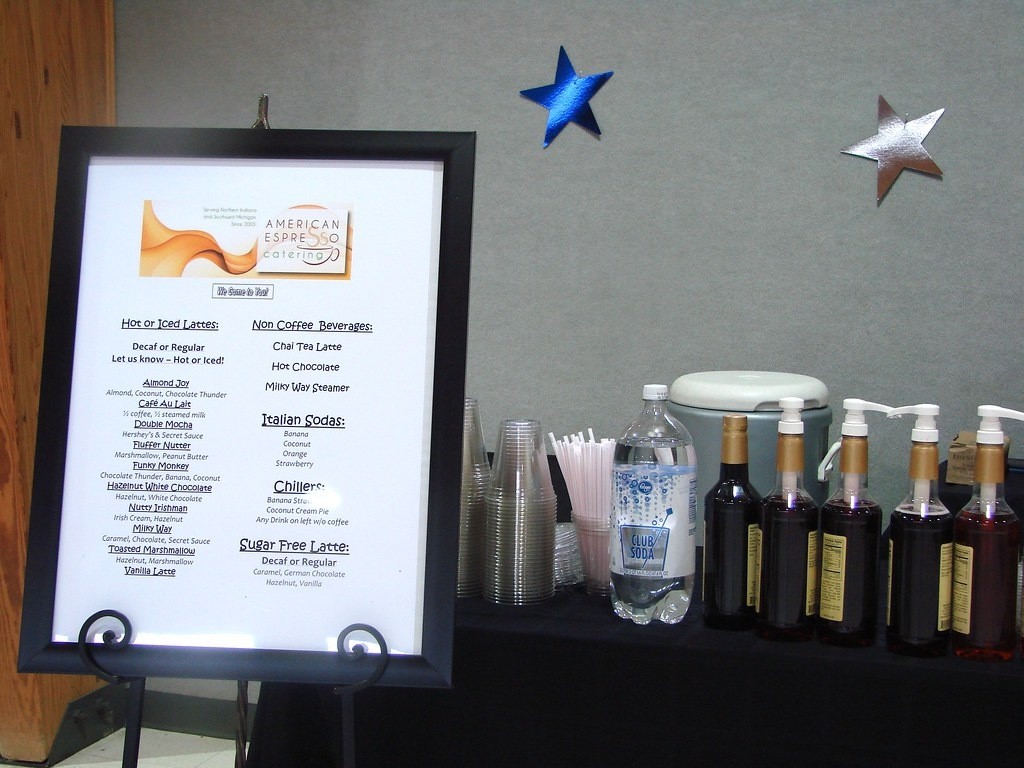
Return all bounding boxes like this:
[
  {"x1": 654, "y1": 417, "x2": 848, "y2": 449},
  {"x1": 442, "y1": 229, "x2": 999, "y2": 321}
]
[
  {"x1": 456, "y1": 399, "x2": 557, "y2": 607},
  {"x1": 572, "y1": 511, "x2": 610, "y2": 598}
]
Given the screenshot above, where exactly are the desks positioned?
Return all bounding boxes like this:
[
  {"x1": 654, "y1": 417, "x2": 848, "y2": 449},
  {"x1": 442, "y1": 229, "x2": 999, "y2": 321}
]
[{"x1": 247, "y1": 546, "x2": 1024, "y2": 768}]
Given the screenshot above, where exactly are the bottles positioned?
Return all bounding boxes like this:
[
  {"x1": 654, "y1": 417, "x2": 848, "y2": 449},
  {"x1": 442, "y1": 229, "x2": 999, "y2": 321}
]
[
  {"x1": 703, "y1": 414, "x2": 762, "y2": 632},
  {"x1": 820, "y1": 437, "x2": 893, "y2": 649},
  {"x1": 883, "y1": 442, "x2": 954, "y2": 658},
  {"x1": 950, "y1": 445, "x2": 1024, "y2": 649},
  {"x1": 608, "y1": 384, "x2": 698, "y2": 625},
  {"x1": 762, "y1": 434, "x2": 820, "y2": 644}
]
